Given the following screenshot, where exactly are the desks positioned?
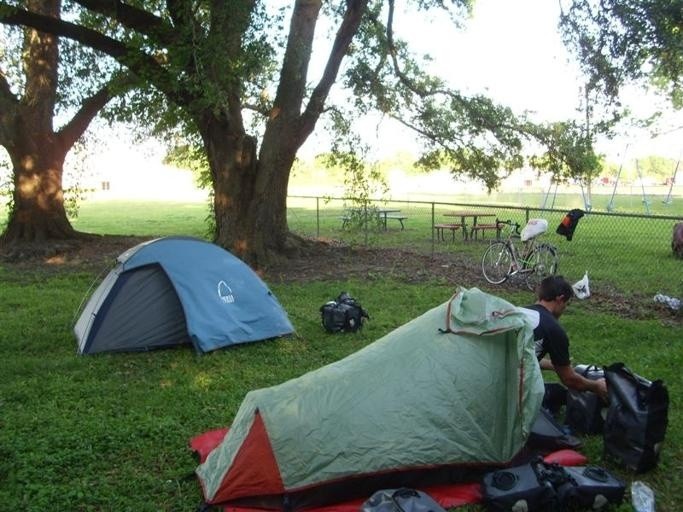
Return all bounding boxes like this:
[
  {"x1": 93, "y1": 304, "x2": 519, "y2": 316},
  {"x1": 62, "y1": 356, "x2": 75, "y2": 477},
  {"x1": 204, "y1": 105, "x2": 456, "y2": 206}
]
[
  {"x1": 441, "y1": 212, "x2": 494, "y2": 241},
  {"x1": 343, "y1": 206, "x2": 400, "y2": 229}
]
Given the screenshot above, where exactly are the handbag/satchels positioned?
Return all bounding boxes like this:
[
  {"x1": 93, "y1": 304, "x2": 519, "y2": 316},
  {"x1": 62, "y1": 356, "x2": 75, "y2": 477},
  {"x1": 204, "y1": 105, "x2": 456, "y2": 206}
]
[
  {"x1": 563, "y1": 355, "x2": 606, "y2": 436},
  {"x1": 602, "y1": 360, "x2": 670, "y2": 475},
  {"x1": 569, "y1": 269, "x2": 591, "y2": 300}
]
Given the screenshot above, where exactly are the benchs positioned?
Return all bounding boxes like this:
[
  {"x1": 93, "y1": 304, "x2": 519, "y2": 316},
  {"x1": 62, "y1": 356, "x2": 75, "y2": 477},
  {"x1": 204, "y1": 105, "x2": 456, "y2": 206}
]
[
  {"x1": 339, "y1": 215, "x2": 407, "y2": 231},
  {"x1": 434, "y1": 222, "x2": 504, "y2": 242}
]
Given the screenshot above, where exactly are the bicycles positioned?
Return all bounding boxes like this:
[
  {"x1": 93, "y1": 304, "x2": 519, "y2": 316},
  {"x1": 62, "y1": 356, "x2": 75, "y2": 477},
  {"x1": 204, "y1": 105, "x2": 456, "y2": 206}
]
[{"x1": 482, "y1": 218, "x2": 557, "y2": 292}]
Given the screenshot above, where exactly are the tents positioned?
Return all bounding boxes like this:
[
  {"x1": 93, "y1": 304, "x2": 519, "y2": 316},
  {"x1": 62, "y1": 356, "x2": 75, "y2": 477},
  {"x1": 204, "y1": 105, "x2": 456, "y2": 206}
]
[
  {"x1": 68, "y1": 234, "x2": 298, "y2": 361},
  {"x1": 185, "y1": 283, "x2": 580, "y2": 511}
]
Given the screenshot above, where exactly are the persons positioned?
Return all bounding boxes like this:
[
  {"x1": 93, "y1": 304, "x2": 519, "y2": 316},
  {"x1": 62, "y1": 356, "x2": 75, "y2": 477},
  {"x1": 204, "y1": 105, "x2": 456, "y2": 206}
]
[{"x1": 518, "y1": 274, "x2": 608, "y2": 416}]
